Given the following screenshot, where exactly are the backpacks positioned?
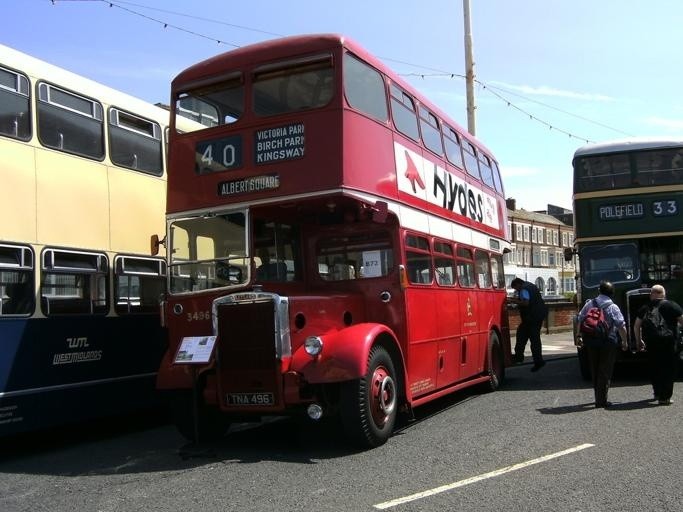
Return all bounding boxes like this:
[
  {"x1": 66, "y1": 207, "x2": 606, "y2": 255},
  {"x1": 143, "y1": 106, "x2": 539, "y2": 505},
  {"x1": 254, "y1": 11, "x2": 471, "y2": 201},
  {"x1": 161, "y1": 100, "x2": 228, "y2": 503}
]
[
  {"x1": 581, "y1": 299, "x2": 612, "y2": 346},
  {"x1": 640, "y1": 304, "x2": 673, "y2": 338}
]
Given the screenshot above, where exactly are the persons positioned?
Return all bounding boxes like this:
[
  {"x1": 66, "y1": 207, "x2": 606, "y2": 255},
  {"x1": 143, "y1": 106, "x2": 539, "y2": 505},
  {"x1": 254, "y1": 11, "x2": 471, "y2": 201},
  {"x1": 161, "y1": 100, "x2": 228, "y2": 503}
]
[
  {"x1": 509, "y1": 277, "x2": 548, "y2": 373},
  {"x1": 572, "y1": 281, "x2": 629, "y2": 408},
  {"x1": 635, "y1": 284, "x2": 682, "y2": 407}
]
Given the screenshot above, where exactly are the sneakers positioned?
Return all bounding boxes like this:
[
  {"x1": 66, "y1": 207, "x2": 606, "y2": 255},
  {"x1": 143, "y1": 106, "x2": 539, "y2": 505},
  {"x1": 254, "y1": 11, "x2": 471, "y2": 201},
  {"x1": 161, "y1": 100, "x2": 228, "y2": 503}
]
[
  {"x1": 659, "y1": 399, "x2": 674, "y2": 404},
  {"x1": 596, "y1": 402, "x2": 611, "y2": 408},
  {"x1": 530, "y1": 360, "x2": 545, "y2": 372}
]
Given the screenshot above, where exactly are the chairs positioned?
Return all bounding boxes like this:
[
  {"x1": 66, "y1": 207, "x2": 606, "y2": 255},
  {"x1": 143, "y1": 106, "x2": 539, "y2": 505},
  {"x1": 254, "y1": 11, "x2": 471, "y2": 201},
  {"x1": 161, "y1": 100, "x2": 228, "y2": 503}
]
[
  {"x1": 255, "y1": 263, "x2": 286, "y2": 282},
  {"x1": 3, "y1": 284, "x2": 35, "y2": 314}
]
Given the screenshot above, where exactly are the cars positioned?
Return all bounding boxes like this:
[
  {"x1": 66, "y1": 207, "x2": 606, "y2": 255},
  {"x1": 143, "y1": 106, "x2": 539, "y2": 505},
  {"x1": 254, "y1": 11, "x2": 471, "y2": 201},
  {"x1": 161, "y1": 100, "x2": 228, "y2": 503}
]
[{"x1": 564, "y1": 139, "x2": 682, "y2": 385}]
